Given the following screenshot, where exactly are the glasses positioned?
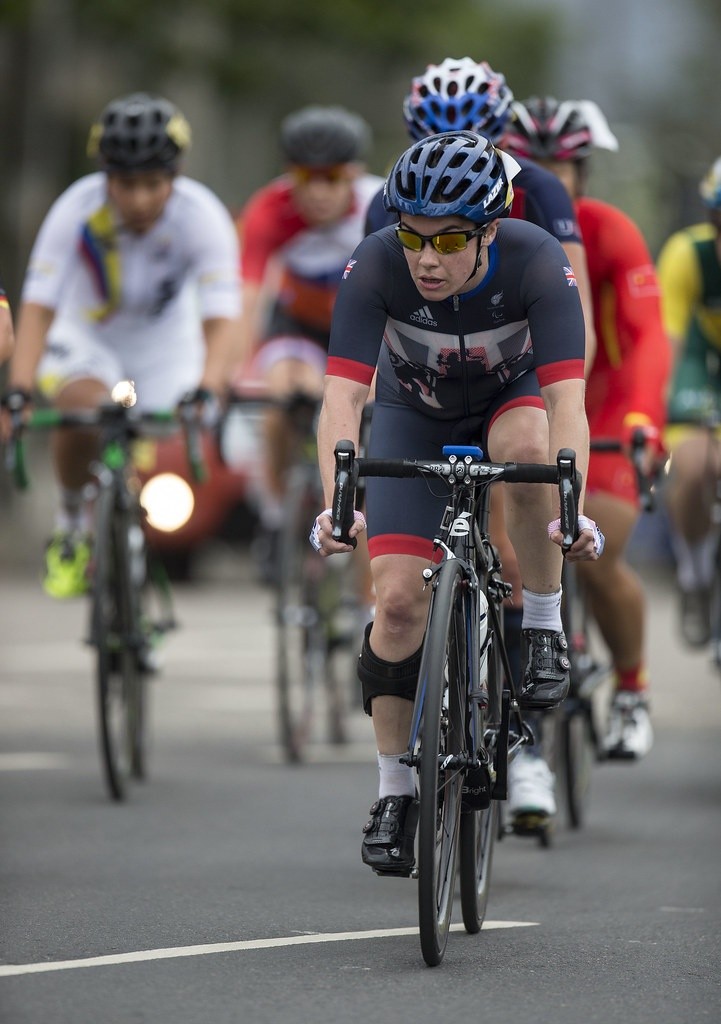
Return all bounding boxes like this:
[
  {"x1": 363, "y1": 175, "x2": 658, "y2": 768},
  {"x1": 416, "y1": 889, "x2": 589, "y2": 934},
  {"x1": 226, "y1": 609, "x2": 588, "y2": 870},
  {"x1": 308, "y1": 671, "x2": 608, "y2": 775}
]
[{"x1": 394, "y1": 219, "x2": 495, "y2": 255}]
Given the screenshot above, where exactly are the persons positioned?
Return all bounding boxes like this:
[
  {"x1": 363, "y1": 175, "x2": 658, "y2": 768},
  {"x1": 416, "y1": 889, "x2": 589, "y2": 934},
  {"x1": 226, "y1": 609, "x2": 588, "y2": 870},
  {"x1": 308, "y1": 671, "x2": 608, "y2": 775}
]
[
  {"x1": 309, "y1": 133, "x2": 605, "y2": 870},
  {"x1": 0, "y1": 286, "x2": 15, "y2": 446},
  {"x1": 222, "y1": 109, "x2": 389, "y2": 644},
  {"x1": 656, "y1": 158, "x2": 721, "y2": 642},
  {"x1": 517, "y1": 97, "x2": 674, "y2": 758},
  {"x1": 364, "y1": 56, "x2": 597, "y2": 818},
  {"x1": 0, "y1": 92, "x2": 240, "y2": 658}
]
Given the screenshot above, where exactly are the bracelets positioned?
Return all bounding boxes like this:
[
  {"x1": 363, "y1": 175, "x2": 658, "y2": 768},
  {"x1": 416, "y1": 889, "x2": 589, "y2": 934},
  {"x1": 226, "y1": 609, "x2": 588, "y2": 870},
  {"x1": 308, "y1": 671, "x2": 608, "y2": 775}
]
[{"x1": 4, "y1": 389, "x2": 27, "y2": 411}]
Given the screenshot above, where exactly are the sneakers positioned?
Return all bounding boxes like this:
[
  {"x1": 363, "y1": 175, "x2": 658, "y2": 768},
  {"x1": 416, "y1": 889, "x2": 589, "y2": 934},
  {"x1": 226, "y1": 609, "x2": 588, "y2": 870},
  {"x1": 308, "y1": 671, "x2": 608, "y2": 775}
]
[
  {"x1": 43, "y1": 529, "x2": 89, "y2": 599},
  {"x1": 599, "y1": 695, "x2": 654, "y2": 757},
  {"x1": 508, "y1": 755, "x2": 556, "y2": 816},
  {"x1": 361, "y1": 785, "x2": 420, "y2": 869},
  {"x1": 517, "y1": 627, "x2": 570, "y2": 708}
]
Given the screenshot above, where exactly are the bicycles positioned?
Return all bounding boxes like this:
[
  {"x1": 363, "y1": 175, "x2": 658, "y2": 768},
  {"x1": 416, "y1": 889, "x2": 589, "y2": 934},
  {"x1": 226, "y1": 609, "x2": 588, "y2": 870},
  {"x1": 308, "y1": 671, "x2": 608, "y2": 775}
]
[
  {"x1": 333, "y1": 433, "x2": 721, "y2": 967},
  {"x1": 9, "y1": 384, "x2": 351, "y2": 802}
]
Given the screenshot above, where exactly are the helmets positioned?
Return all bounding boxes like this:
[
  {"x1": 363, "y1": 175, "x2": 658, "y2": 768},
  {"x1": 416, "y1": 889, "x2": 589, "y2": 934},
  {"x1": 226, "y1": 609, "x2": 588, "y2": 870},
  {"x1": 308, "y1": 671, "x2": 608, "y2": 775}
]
[
  {"x1": 279, "y1": 109, "x2": 368, "y2": 163},
  {"x1": 700, "y1": 157, "x2": 720, "y2": 210},
  {"x1": 383, "y1": 131, "x2": 521, "y2": 225},
  {"x1": 508, "y1": 97, "x2": 592, "y2": 160},
  {"x1": 402, "y1": 57, "x2": 514, "y2": 145},
  {"x1": 87, "y1": 95, "x2": 191, "y2": 165}
]
